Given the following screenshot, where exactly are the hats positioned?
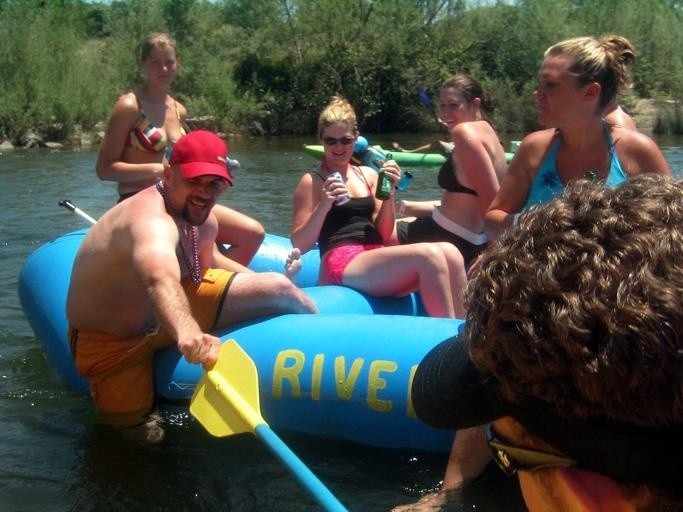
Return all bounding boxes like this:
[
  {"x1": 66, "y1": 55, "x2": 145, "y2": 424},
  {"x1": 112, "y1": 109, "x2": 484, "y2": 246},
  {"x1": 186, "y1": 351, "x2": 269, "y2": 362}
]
[
  {"x1": 169, "y1": 129, "x2": 234, "y2": 186},
  {"x1": 411, "y1": 333, "x2": 644, "y2": 480}
]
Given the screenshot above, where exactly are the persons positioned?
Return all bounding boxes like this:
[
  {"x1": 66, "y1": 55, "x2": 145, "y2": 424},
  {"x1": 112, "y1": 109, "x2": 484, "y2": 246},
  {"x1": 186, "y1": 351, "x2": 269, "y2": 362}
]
[
  {"x1": 93, "y1": 31, "x2": 302, "y2": 283},
  {"x1": 64, "y1": 124, "x2": 319, "y2": 446},
  {"x1": 287, "y1": 91, "x2": 468, "y2": 320},
  {"x1": 387, "y1": 33, "x2": 672, "y2": 511},
  {"x1": 387, "y1": 70, "x2": 509, "y2": 268},
  {"x1": 390, "y1": 138, "x2": 455, "y2": 154},
  {"x1": 410, "y1": 168, "x2": 681, "y2": 509},
  {"x1": 604, "y1": 104, "x2": 637, "y2": 133}
]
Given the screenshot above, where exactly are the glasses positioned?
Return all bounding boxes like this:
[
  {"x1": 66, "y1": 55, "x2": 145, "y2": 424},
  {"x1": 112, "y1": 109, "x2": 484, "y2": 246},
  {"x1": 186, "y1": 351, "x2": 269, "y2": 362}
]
[
  {"x1": 485, "y1": 426, "x2": 594, "y2": 478},
  {"x1": 321, "y1": 135, "x2": 353, "y2": 147}
]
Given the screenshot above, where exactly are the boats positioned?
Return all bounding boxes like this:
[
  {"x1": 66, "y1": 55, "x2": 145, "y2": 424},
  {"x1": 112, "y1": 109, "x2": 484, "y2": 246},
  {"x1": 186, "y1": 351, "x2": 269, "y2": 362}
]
[
  {"x1": 16, "y1": 225, "x2": 500, "y2": 466},
  {"x1": 302, "y1": 140, "x2": 522, "y2": 170}
]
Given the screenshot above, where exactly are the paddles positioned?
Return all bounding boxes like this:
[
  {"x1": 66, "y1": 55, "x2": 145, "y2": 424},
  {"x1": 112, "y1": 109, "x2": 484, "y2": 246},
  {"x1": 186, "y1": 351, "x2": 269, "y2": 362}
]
[{"x1": 188, "y1": 338, "x2": 348, "y2": 512}]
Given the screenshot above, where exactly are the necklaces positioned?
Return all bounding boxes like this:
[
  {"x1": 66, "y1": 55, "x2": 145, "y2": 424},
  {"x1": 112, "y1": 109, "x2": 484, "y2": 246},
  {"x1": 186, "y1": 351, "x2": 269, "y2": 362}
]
[{"x1": 153, "y1": 179, "x2": 204, "y2": 290}]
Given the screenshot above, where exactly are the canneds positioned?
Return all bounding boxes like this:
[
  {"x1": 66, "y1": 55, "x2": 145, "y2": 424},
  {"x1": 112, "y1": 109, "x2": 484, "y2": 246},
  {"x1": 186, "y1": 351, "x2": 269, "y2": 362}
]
[{"x1": 325, "y1": 172, "x2": 351, "y2": 207}]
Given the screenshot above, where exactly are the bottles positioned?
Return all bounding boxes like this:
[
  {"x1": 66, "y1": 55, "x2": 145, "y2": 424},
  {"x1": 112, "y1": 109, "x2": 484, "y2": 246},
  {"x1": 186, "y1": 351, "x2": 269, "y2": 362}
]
[
  {"x1": 584, "y1": 169, "x2": 598, "y2": 184},
  {"x1": 375, "y1": 153, "x2": 393, "y2": 200}
]
